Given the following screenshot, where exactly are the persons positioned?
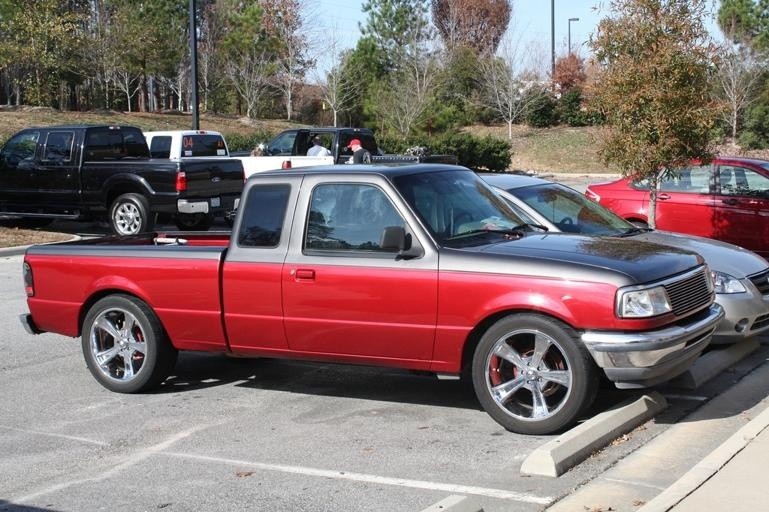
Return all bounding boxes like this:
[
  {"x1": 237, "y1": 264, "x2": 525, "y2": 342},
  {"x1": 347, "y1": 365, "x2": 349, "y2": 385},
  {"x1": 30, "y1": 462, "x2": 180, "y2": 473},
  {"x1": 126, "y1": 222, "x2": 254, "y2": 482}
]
[
  {"x1": 305, "y1": 136, "x2": 331, "y2": 155},
  {"x1": 344, "y1": 138, "x2": 372, "y2": 165}
]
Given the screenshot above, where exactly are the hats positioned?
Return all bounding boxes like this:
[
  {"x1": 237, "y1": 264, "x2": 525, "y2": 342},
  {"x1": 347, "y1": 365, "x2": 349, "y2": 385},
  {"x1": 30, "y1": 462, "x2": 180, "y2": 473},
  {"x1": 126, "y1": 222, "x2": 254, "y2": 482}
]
[{"x1": 346, "y1": 139, "x2": 362, "y2": 148}]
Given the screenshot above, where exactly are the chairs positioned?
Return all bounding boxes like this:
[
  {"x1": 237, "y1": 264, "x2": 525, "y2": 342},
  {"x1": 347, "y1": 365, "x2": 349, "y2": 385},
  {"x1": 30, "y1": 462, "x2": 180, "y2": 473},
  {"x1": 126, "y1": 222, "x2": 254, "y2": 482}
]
[{"x1": 661, "y1": 167, "x2": 749, "y2": 195}]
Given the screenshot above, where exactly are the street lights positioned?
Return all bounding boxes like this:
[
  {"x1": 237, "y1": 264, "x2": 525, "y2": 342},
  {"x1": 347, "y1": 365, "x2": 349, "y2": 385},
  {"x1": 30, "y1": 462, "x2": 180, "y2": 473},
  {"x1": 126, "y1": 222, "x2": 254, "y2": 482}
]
[{"x1": 568, "y1": 19, "x2": 578, "y2": 56}]
